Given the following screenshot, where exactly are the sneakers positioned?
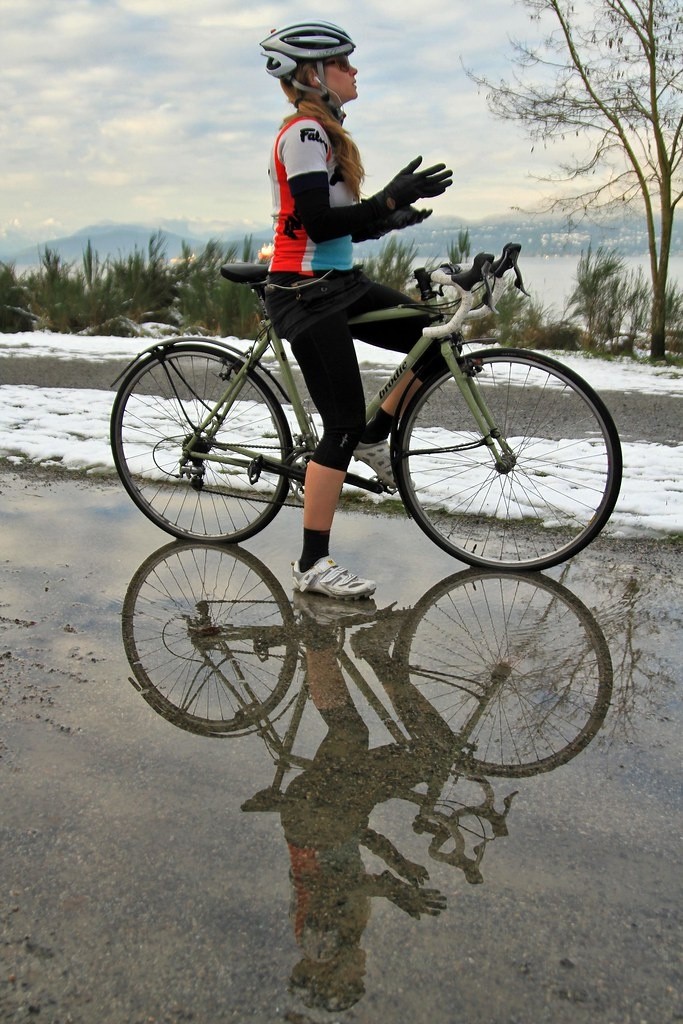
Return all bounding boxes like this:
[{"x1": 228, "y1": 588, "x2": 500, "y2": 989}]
[
  {"x1": 352, "y1": 439, "x2": 416, "y2": 490},
  {"x1": 291, "y1": 555, "x2": 376, "y2": 601}
]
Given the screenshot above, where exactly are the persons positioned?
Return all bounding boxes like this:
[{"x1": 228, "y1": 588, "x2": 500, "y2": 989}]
[
  {"x1": 259, "y1": 20, "x2": 463, "y2": 601},
  {"x1": 281, "y1": 591, "x2": 457, "y2": 1013}
]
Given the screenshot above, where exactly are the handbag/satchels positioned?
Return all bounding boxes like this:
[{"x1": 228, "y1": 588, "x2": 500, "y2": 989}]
[{"x1": 296, "y1": 278, "x2": 347, "y2": 312}]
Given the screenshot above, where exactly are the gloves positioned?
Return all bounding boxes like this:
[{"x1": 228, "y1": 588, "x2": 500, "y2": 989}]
[
  {"x1": 373, "y1": 155, "x2": 453, "y2": 217},
  {"x1": 383, "y1": 204, "x2": 433, "y2": 229}
]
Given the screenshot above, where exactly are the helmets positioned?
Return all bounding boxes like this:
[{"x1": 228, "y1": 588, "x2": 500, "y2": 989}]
[{"x1": 259, "y1": 20, "x2": 356, "y2": 80}]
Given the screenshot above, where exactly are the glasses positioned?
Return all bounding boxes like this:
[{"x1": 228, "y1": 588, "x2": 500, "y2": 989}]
[{"x1": 311, "y1": 54, "x2": 350, "y2": 72}]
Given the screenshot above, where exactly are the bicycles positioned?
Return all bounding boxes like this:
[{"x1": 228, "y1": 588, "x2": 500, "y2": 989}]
[
  {"x1": 110, "y1": 242, "x2": 622, "y2": 575},
  {"x1": 120, "y1": 542, "x2": 613, "y2": 883}
]
